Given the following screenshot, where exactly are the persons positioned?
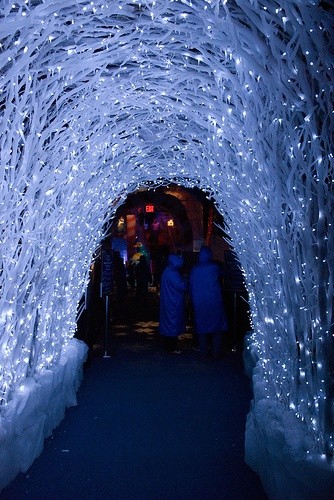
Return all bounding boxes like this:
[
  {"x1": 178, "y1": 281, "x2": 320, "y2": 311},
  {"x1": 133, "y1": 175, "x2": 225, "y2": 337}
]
[
  {"x1": 129, "y1": 230, "x2": 153, "y2": 304},
  {"x1": 188, "y1": 247, "x2": 228, "y2": 362},
  {"x1": 158, "y1": 252, "x2": 189, "y2": 354},
  {"x1": 111, "y1": 226, "x2": 130, "y2": 298}
]
